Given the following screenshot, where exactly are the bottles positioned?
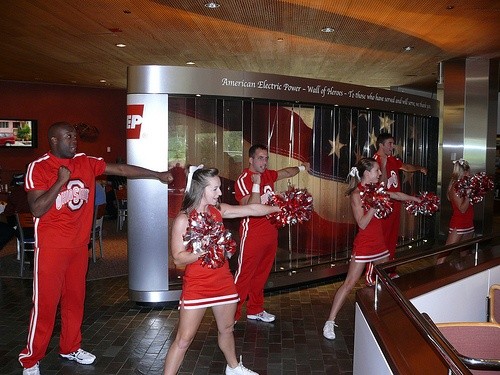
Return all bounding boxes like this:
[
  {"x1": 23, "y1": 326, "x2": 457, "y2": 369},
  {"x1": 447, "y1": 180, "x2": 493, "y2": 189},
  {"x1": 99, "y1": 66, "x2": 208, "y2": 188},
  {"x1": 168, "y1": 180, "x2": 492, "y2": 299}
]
[{"x1": 0, "y1": 183, "x2": 9, "y2": 193}]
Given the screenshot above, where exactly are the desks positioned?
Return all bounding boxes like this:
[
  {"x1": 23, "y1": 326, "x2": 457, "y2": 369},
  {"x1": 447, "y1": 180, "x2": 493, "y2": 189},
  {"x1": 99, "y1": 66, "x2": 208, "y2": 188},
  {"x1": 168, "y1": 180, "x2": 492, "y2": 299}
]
[{"x1": 0, "y1": 185, "x2": 31, "y2": 217}]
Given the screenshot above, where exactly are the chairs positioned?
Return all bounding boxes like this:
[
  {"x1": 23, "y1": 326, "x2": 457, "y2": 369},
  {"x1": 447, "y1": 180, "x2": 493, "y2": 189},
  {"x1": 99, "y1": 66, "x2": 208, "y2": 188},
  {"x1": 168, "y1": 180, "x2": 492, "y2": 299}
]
[
  {"x1": 90, "y1": 203, "x2": 107, "y2": 263},
  {"x1": 16, "y1": 209, "x2": 36, "y2": 277},
  {"x1": 115, "y1": 187, "x2": 128, "y2": 233}
]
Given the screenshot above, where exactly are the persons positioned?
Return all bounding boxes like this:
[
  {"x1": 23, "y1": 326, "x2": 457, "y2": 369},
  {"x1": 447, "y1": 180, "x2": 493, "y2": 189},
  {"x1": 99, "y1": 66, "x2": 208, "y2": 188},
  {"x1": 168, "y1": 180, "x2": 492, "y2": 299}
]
[
  {"x1": 372, "y1": 133, "x2": 429, "y2": 280},
  {"x1": 162, "y1": 165, "x2": 282, "y2": 375},
  {"x1": 437, "y1": 159, "x2": 475, "y2": 271},
  {"x1": 324, "y1": 157, "x2": 423, "y2": 340},
  {"x1": 232, "y1": 144, "x2": 312, "y2": 322},
  {"x1": 18, "y1": 121, "x2": 174, "y2": 375}
]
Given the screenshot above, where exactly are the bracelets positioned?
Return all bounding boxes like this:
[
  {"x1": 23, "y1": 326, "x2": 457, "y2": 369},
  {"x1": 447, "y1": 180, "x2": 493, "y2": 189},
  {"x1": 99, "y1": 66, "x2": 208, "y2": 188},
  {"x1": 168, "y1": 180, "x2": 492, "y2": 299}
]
[
  {"x1": 297, "y1": 165, "x2": 306, "y2": 172},
  {"x1": 252, "y1": 184, "x2": 261, "y2": 193}
]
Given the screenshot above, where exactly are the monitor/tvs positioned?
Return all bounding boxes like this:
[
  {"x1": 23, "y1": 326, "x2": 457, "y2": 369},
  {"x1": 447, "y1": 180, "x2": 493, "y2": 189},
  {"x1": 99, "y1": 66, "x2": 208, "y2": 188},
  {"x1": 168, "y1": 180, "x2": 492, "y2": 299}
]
[{"x1": 0, "y1": 118, "x2": 38, "y2": 149}]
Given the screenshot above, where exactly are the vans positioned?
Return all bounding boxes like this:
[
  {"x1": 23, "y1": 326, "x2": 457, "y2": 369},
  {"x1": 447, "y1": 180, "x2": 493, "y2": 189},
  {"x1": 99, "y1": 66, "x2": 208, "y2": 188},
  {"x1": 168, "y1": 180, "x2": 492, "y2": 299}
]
[{"x1": 0, "y1": 132, "x2": 15, "y2": 146}]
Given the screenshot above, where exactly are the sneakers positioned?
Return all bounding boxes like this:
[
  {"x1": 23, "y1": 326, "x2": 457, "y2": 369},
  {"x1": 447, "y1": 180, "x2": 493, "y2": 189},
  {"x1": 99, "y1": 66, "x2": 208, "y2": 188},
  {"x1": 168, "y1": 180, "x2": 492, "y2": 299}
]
[
  {"x1": 60, "y1": 348, "x2": 96, "y2": 364},
  {"x1": 323, "y1": 320, "x2": 339, "y2": 340},
  {"x1": 225, "y1": 355, "x2": 260, "y2": 375},
  {"x1": 23, "y1": 361, "x2": 40, "y2": 375},
  {"x1": 247, "y1": 310, "x2": 276, "y2": 323}
]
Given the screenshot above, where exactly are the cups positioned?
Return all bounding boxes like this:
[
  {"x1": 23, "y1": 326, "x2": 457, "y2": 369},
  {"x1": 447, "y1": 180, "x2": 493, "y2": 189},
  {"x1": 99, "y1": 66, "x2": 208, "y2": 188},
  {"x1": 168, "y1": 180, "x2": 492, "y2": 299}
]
[{"x1": 118, "y1": 185, "x2": 125, "y2": 191}]
